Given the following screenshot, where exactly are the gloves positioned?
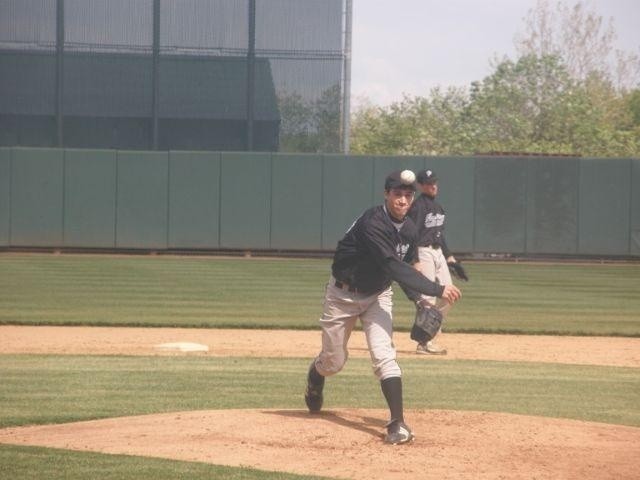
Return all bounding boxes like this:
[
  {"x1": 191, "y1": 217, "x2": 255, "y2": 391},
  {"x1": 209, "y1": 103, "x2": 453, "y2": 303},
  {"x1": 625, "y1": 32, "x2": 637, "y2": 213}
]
[{"x1": 447, "y1": 260, "x2": 469, "y2": 281}]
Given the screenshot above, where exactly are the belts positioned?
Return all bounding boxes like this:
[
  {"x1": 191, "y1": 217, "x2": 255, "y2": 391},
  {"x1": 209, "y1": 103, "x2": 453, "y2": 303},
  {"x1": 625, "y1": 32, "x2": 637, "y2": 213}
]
[
  {"x1": 336, "y1": 280, "x2": 366, "y2": 293},
  {"x1": 417, "y1": 243, "x2": 440, "y2": 248}
]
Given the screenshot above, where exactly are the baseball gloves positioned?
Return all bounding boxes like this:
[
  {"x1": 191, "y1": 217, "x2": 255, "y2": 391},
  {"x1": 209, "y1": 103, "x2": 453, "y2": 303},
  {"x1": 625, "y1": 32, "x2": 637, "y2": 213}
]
[
  {"x1": 411, "y1": 304, "x2": 443, "y2": 343},
  {"x1": 447, "y1": 262, "x2": 468, "y2": 282}
]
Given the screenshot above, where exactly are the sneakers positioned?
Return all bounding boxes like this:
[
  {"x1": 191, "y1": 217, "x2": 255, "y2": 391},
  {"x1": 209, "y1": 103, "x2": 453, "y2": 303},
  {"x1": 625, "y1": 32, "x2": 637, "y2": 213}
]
[
  {"x1": 417, "y1": 343, "x2": 446, "y2": 356},
  {"x1": 385, "y1": 420, "x2": 411, "y2": 445},
  {"x1": 305, "y1": 363, "x2": 324, "y2": 411}
]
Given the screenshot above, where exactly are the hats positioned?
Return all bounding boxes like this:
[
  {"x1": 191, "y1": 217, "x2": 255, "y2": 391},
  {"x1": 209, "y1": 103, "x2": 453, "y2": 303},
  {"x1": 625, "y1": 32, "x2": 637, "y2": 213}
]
[
  {"x1": 417, "y1": 169, "x2": 438, "y2": 184},
  {"x1": 386, "y1": 170, "x2": 415, "y2": 193}
]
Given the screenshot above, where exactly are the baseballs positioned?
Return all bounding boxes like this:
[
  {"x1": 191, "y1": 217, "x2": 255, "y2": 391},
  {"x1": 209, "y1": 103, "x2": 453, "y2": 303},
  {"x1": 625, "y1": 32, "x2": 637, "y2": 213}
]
[{"x1": 401, "y1": 170, "x2": 416, "y2": 185}]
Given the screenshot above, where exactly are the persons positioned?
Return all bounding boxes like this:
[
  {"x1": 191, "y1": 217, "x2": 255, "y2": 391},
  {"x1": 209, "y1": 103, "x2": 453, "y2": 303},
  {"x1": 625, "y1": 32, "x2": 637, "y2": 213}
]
[
  {"x1": 405, "y1": 167, "x2": 469, "y2": 356},
  {"x1": 303, "y1": 168, "x2": 463, "y2": 446}
]
